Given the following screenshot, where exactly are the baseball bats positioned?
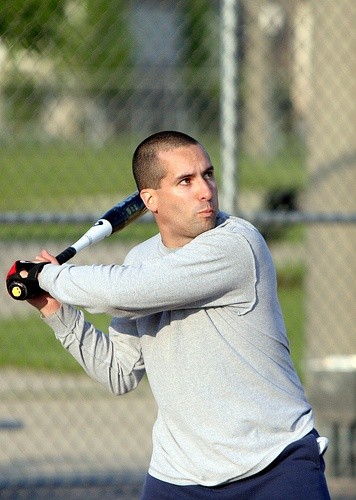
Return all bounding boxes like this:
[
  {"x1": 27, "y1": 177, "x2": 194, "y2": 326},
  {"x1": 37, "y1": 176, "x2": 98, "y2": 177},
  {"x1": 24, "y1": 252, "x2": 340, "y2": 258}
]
[{"x1": 10, "y1": 190, "x2": 149, "y2": 300}]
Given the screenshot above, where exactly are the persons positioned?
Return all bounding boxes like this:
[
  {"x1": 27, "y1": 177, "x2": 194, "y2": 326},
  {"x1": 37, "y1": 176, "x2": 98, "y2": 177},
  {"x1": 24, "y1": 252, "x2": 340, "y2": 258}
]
[{"x1": 9, "y1": 129, "x2": 336, "y2": 500}]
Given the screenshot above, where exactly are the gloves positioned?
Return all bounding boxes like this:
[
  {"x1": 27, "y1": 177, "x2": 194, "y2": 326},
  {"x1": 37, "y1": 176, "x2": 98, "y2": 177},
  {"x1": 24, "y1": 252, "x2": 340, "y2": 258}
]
[{"x1": 4, "y1": 259, "x2": 47, "y2": 300}]
